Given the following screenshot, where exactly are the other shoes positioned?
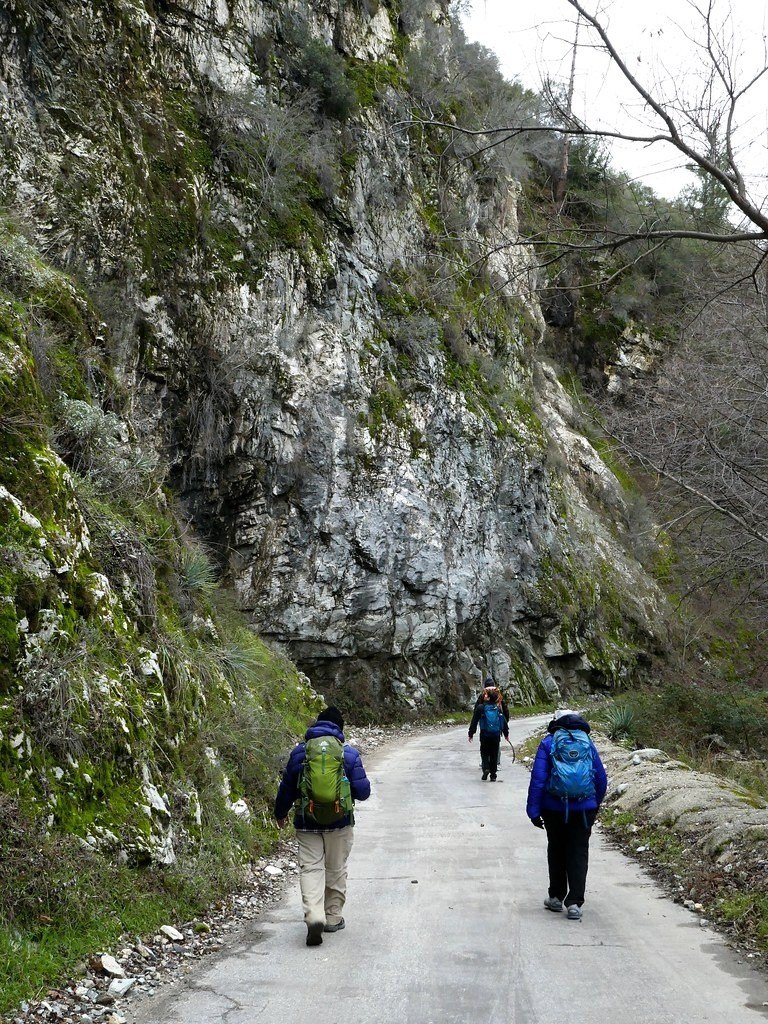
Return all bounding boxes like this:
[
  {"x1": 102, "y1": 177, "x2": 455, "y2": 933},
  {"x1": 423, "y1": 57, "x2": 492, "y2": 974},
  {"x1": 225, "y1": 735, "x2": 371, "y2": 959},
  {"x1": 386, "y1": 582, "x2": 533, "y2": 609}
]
[
  {"x1": 478, "y1": 762, "x2": 482, "y2": 769},
  {"x1": 490, "y1": 774, "x2": 497, "y2": 781},
  {"x1": 481, "y1": 769, "x2": 489, "y2": 780},
  {"x1": 497, "y1": 763, "x2": 504, "y2": 771}
]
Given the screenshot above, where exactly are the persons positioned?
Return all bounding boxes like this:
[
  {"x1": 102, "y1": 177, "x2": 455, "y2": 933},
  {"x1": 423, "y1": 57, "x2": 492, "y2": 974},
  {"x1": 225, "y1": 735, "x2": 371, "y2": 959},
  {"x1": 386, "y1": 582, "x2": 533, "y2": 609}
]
[
  {"x1": 467, "y1": 691, "x2": 510, "y2": 781},
  {"x1": 525, "y1": 709, "x2": 607, "y2": 920},
  {"x1": 274, "y1": 706, "x2": 370, "y2": 947},
  {"x1": 473, "y1": 677, "x2": 509, "y2": 770}
]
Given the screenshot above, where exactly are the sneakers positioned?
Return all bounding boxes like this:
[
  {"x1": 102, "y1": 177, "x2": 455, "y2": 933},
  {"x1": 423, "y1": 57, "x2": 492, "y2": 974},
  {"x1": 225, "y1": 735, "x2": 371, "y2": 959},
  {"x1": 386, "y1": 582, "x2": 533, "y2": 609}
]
[
  {"x1": 544, "y1": 896, "x2": 563, "y2": 911},
  {"x1": 306, "y1": 922, "x2": 324, "y2": 946},
  {"x1": 566, "y1": 904, "x2": 583, "y2": 919},
  {"x1": 324, "y1": 917, "x2": 345, "y2": 932}
]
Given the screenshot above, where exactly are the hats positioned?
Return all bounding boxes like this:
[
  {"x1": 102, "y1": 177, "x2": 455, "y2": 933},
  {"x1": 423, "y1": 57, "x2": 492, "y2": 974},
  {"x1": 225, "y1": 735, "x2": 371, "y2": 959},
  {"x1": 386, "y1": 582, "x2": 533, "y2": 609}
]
[
  {"x1": 317, "y1": 706, "x2": 344, "y2": 730},
  {"x1": 553, "y1": 709, "x2": 579, "y2": 721}
]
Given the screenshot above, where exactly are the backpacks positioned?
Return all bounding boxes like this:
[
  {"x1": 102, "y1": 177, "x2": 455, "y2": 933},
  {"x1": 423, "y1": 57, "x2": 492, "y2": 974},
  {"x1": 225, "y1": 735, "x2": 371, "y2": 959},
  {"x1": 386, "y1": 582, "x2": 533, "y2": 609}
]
[
  {"x1": 296, "y1": 735, "x2": 354, "y2": 828},
  {"x1": 547, "y1": 727, "x2": 597, "y2": 801},
  {"x1": 479, "y1": 702, "x2": 505, "y2": 737}
]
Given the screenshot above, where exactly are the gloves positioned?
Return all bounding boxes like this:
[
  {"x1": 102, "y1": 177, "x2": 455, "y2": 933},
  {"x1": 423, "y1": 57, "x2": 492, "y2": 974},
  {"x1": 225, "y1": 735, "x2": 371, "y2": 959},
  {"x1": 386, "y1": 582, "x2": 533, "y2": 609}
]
[{"x1": 531, "y1": 816, "x2": 545, "y2": 829}]
